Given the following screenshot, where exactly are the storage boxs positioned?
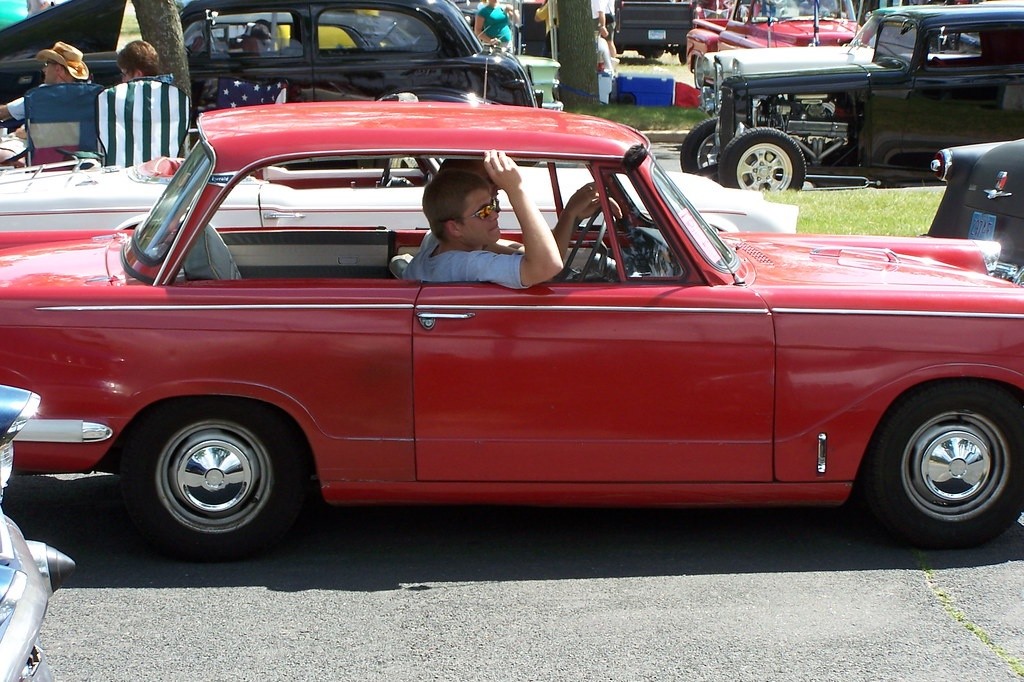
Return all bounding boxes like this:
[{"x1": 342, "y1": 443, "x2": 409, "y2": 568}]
[{"x1": 513, "y1": 55, "x2": 675, "y2": 111}]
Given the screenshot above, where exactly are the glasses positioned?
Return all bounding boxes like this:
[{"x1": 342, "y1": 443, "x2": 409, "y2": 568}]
[
  {"x1": 44, "y1": 61, "x2": 53, "y2": 67},
  {"x1": 455, "y1": 195, "x2": 500, "y2": 222},
  {"x1": 119, "y1": 69, "x2": 137, "y2": 77}
]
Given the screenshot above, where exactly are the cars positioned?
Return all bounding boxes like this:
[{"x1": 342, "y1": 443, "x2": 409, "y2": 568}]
[
  {"x1": 923, "y1": 137, "x2": 1024, "y2": 288},
  {"x1": 184, "y1": 13, "x2": 415, "y2": 49},
  {"x1": 0, "y1": 102, "x2": 1024, "y2": 561},
  {"x1": 0, "y1": 0, "x2": 545, "y2": 170},
  {"x1": 0, "y1": 384, "x2": 76, "y2": 682},
  {"x1": 680, "y1": 5, "x2": 1024, "y2": 191},
  {"x1": 515, "y1": 54, "x2": 566, "y2": 113}
]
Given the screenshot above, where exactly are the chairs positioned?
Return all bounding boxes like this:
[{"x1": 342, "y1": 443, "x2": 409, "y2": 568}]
[
  {"x1": 24, "y1": 77, "x2": 289, "y2": 172},
  {"x1": 183, "y1": 223, "x2": 241, "y2": 281}
]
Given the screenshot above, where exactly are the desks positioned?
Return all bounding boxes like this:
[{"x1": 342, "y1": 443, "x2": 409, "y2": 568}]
[{"x1": 0, "y1": 136, "x2": 27, "y2": 163}]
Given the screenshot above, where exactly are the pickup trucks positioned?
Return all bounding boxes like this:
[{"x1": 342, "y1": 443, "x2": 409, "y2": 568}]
[
  {"x1": 688, "y1": 0, "x2": 866, "y2": 90},
  {"x1": 614, "y1": 1, "x2": 695, "y2": 65}
]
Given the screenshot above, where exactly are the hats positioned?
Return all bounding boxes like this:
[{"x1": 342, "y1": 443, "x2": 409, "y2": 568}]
[
  {"x1": 237, "y1": 22, "x2": 271, "y2": 42},
  {"x1": 35, "y1": 41, "x2": 89, "y2": 80}
]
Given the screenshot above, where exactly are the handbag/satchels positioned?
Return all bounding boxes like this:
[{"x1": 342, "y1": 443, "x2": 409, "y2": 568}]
[{"x1": 606, "y1": 14, "x2": 613, "y2": 23}]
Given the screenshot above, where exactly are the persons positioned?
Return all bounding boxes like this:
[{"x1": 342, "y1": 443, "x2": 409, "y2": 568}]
[
  {"x1": 251, "y1": 19, "x2": 278, "y2": 51},
  {"x1": 116, "y1": 40, "x2": 174, "y2": 84},
  {"x1": 738, "y1": 3, "x2": 749, "y2": 19},
  {"x1": 603, "y1": 0, "x2": 616, "y2": 58},
  {"x1": 591, "y1": 0, "x2": 613, "y2": 77},
  {"x1": 475, "y1": 0, "x2": 513, "y2": 54},
  {"x1": 420, "y1": 159, "x2": 623, "y2": 262},
  {"x1": 535, "y1": 0, "x2": 559, "y2": 53},
  {"x1": 0, "y1": 41, "x2": 89, "y2": 122},
  {"x1": 404, "y1": 149, "x2": 563, "y2": 289}
]
